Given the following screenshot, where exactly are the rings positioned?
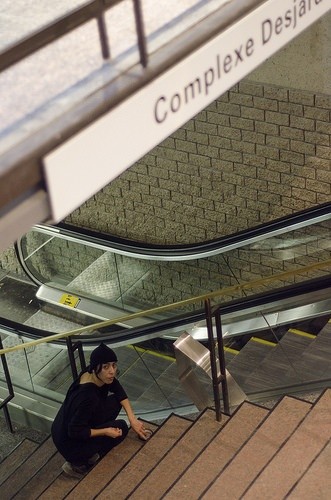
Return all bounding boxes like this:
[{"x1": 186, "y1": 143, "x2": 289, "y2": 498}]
[{"x1": 143, "y1": 427, "x2": 146, "y2": 430}]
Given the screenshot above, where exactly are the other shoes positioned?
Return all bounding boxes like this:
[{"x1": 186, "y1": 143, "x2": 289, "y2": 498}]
[{"x1": 61, "y1": 452, "x2": 99, "y2": 481}]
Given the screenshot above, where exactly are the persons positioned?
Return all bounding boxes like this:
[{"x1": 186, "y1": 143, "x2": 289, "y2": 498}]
[{"x1": 50, "y1": 342, "x2": 154, "y2": 481}]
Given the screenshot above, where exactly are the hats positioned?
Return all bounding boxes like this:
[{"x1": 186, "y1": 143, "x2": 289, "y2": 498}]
[{"x1": 87, "y1": 342, "x2": 117, "y2": 370}]
